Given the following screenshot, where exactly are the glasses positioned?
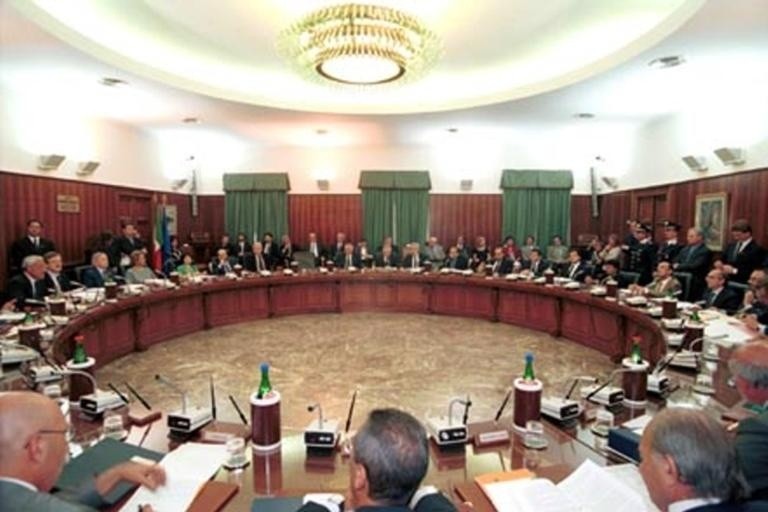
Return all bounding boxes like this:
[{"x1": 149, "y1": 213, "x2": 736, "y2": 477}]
[{"x1": 24, "y1": 424, "x2": 76, "y2": 450}]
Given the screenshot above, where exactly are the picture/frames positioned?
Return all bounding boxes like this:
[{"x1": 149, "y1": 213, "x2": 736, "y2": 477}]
[{"x1": 694, "y1": 193, "x2": 729, "y2": 252}]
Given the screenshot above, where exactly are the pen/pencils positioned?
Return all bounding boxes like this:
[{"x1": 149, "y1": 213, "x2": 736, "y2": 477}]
[{"x1": 454, "y1": 487, "x2": 474, "y2": 509}]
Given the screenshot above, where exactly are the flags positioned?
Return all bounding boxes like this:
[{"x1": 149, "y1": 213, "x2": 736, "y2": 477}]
[
  {"x1": 148, "y1": 192, "x2": 161, "y2": 277},
  {"x1": 157, "y1": 194, "x2": 173, "y2": 275}
]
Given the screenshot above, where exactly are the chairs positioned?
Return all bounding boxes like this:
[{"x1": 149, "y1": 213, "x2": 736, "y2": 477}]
[{"x1": 1, "y1": 249, "x2": 767, "y2": 511}]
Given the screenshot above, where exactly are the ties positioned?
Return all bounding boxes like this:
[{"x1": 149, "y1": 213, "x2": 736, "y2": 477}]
[
  {"x1": 413, "y1": 254, "x2": 416, "y2": 268},
  {"x1": 258, "y1": 255, "x2": 263, "y2": 270},
  {"x1": 735, "y1": 241, "x2": 741, "y2": 255}
]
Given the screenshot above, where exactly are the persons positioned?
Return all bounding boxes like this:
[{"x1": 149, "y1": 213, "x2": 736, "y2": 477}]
[{"x1": 0, "y1": 217, "x2": 766, "y2": 512}]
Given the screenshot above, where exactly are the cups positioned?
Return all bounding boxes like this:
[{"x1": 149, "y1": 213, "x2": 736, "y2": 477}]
[
  {"x1": 105, "y1": 416, "x2": 124, "y2": 440},
  {"x1": 623, "y1": 403, "x2": 644, "y2": 425},
  {"x1": 524, "y1": 421, "x2": 545, "y2": 446},
  {"x1": 509, "y1": 432, "x2": 537, "y2": 472},
  {"x1": 18, "y1": 324, "x2": 41, "y2": 351},
  {"x1": 661, "y1": 300, "x2": 678, "y2": 318},
  {"x1": 684, "y1": 319, "x2": 704, "y2": 354},
  {"x1": 66, "y1": 357, "x2": 97, "y2": 403},
  {"x1": 249, "y1": 390, "x2": 281, "y2": 449},
  {"x1": 224, "y1": 436, "x2": 246, "y2": 465},
  {"x1": 67, "y1": 401, "x2": 99, "y2": 443},
  {"x1": 512, "y1": 375, "x2": 542, "y2": 429},
  {"x1": 622, "y1": 358, "x2": 649, "y2": 404},
  {"x1": 44, "y1": 247, "x2": 380, "y2": 313},
  {"x1": 594, "y1": 409, "x2": 615, "y2": 431},
  {"x1": 251, "y1": 447, "x2": 283, "y2": 497},
  {"x1": 421, "y1": 259, "x2": 618, "y2": 299}
]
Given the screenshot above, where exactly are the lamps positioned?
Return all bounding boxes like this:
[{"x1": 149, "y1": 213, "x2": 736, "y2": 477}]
[{"x1": 277, "y1": 2, "x2": 440, "y2": 90}]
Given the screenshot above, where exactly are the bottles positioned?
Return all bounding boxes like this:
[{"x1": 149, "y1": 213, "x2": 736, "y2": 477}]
[
  {"x1": 71, "y1": 334, "x2": 91, "y2": 361},
  {"x1": 628, "y1": 334, "x2": 643, "y2": 364},
  {"x1": 256, "y1": 361, "x2": 273, "y2": 399},
  {"x1": 22, "y1": 306, "x2": 36, "y2": 327},
  {"x1": 521, "y1": 352, "x2": 537, "y2": 384},
  {"x1": 688, "y1": 304, "x2": 702, "y2": 326},
  {"x1": 666, "y1": 284, "x2": 674, "y2": 299}
]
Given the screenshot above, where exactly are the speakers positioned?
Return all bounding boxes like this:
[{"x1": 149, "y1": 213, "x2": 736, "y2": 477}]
[
  {"x1": 682, "y1": 156, "x2": 709, "y2": 172},
  {"x1": 317, "y1": 179, "x2": 330, "y2": 191},
  {"x1": 714, "y1": 147, "x2": 745, "y2": 166},
  {"x1": 172, "y1": 179, "x2": 189, "y2": 191},
  {"x1": 460, "y1": 180, "x2": 474, "y2": 192},
  {"x1": 602, "y1": 176, "x2": 620, "y2": 189},
  {"x1": 76, "y1": 159, "x2": 101, "y2": 176},
  {"x1": 39, "y1": 153, "x2": 66, "y2": 171}
]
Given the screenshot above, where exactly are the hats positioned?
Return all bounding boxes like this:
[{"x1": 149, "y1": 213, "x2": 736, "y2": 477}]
[{"x1": 662, "y1": 219, "x2": 681, "y2": 232}]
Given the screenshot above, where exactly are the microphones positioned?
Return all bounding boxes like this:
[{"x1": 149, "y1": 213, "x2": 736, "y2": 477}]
[
  {"x1": 70, "y1": 280, "x2": 106, "y2": 307},
  {"x1": 154, "y1": 268, "x2": 175, "y2": 291},
  {"x1": 114, "y1": 274, "x2": 141, "y2": 296},
  {"x1": 562, "y1": 269, "x2": 708, "y2": 319},
  {"x1": 667, "y1": 315, "x2": 721, "y2": 347},
  {"x1": 580, "y1": 365, "x2": 650, "y2": 407},
  {"x1": 663, "y1": 333, "x2": 730, "y2": 374},
  {"x1": 646, "y1": 346, "x2": 684, "y2": 394},
  {"x1": 47, "y1": 287, "x2": 88, "y2": 312},
  {"x1": 24, "y1": 298, "x2": 70, "y2": 326},
  {"x1": 540, "y1": 376, "x2": 599, "y2": 422},
  {"x1": 50, "y1": 370, "x2": 129, "y2": 415},
  {"x1": 303, "y1": 402, "x2": 341, "y2": 448},
  {"x1": 154, "y1": 373, "x2": 214, "y2": 433},
  {"x1": 426, "y1": 398, "x2": 472, "y2": 446}
]
